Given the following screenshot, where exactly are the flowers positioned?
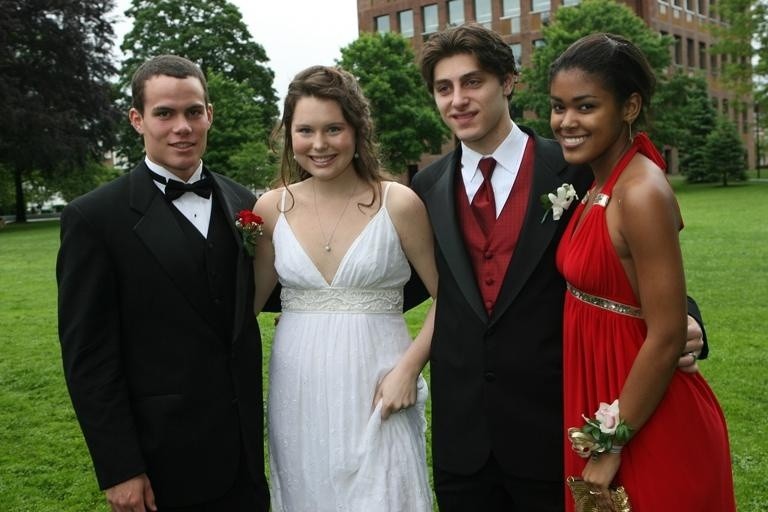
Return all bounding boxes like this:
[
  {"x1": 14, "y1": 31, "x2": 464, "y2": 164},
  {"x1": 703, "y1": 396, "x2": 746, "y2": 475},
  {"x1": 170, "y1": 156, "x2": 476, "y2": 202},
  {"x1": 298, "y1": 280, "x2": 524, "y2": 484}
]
[
  {"x1": 233, "y1": 208, "x2": 267, "y2": 260},
  {"x1": 539, "y1": 181, "x2": 580, "y2": 226}
]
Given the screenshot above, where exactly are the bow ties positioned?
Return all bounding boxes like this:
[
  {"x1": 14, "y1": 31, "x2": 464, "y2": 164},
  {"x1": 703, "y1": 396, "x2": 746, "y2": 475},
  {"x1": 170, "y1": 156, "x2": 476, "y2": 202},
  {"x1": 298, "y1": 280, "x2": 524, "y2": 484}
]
[{"x1": 164, "y1": 178, "x2": 210, "y2": 202}]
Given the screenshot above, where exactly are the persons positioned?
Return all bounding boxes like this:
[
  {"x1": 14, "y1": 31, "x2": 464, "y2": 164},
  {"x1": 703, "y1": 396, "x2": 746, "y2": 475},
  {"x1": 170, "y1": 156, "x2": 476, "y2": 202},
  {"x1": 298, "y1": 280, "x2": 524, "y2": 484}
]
[
  {"x1": 55, "y1": 51, "x2": 283, "y2": 511},
  {"x1": 543, "y1": 29, "x2": 737, "y2": 512},
  {"x1": 393, "y1": 20, "x2": 707, "y2": 512},
  {"x1": 251, "y1": 64, "x2": 440, "y2": 512}
]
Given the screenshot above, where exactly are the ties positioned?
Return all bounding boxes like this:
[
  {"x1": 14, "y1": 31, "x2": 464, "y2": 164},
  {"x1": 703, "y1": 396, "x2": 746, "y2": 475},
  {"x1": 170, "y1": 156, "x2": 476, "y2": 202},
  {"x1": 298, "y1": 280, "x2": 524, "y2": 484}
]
[{"x1": 470, "y1": 157, "x2": 496, "y2": 230}]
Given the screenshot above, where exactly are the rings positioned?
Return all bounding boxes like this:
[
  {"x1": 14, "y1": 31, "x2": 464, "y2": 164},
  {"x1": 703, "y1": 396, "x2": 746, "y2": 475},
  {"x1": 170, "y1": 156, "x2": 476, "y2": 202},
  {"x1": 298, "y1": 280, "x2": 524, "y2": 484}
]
[
  {"x1": 688, "y1": 352, "x2": 697, "y2": 364},
  {"x1": 595, "y1": 489, "x2": 602, "y2": 496},
  {"x1": 589, "y1": 490, "x2": 596, "y2": 496}
]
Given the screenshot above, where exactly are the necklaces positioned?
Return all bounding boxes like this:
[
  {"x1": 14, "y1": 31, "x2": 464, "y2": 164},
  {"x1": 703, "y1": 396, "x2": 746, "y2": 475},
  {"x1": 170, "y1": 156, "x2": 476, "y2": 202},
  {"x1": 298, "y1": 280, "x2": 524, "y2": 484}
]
[{"x1": 311, "y1": 174, "x2": 361, "y2": 252}]
